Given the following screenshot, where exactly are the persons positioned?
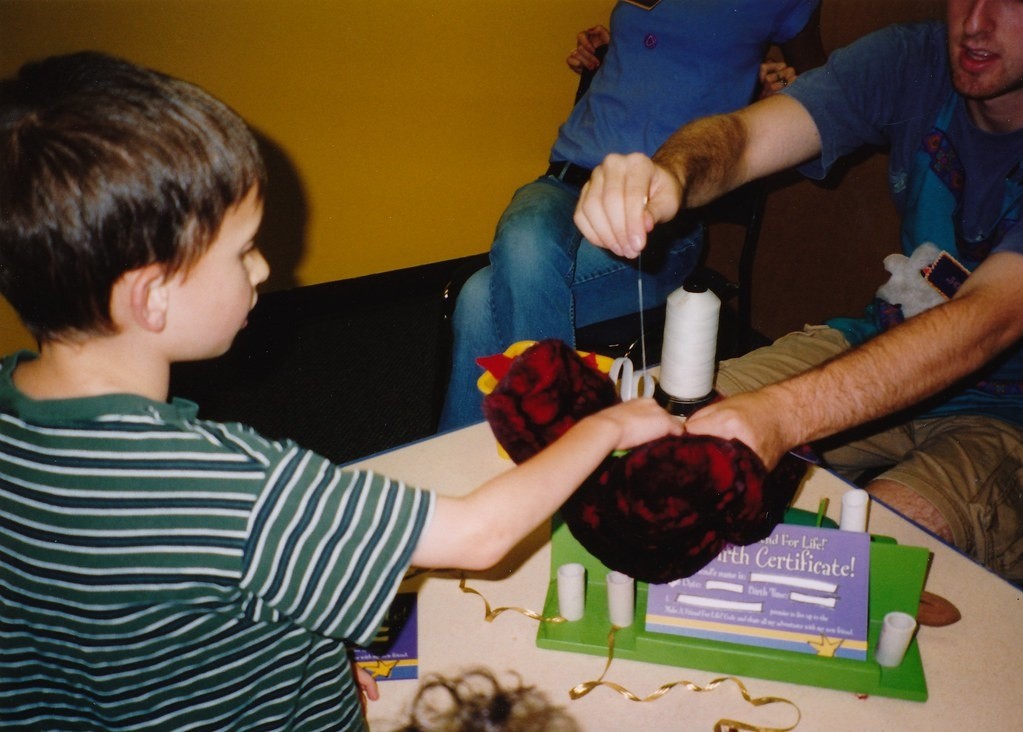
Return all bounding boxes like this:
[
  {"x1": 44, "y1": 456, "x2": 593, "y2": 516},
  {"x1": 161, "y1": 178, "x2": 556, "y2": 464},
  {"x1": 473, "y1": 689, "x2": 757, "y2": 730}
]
[
  {"x1": 573, "y1": 0, "x2": 1023, "y2": 582},
  {"x1": 1, "y1": 51, "x2": 685, "y2": 731},
  {"x1": 435, "y1": 1, "x2": 828, "y2": 430}
]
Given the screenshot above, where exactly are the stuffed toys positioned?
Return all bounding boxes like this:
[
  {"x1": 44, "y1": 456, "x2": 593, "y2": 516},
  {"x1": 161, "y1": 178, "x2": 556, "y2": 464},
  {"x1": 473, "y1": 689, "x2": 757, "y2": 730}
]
[{"x1": 477, "y1": 338, "x2": 808, "y2": 586}]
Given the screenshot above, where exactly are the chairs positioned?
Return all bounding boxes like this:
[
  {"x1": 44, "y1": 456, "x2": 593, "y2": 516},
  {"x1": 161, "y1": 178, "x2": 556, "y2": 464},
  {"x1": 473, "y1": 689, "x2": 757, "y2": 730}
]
[{"x1": 694, "y1": 0, "x2": 949, "y2": 368}]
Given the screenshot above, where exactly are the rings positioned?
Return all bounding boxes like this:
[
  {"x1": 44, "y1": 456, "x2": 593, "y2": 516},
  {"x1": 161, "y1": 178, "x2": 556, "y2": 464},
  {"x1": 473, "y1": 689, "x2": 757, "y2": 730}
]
[
  {"x1": 781, "y1": 79, "x2": 788, "y2": 86},
  {"x1": 777, "y1": 72, "x2": 780, "y2": 80}
]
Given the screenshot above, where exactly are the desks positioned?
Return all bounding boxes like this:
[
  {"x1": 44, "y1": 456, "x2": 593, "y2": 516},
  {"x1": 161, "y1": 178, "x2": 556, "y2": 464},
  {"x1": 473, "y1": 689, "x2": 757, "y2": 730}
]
[{"x1": 334, "y1": 422, "x2": 1023, "y2": 732}]
